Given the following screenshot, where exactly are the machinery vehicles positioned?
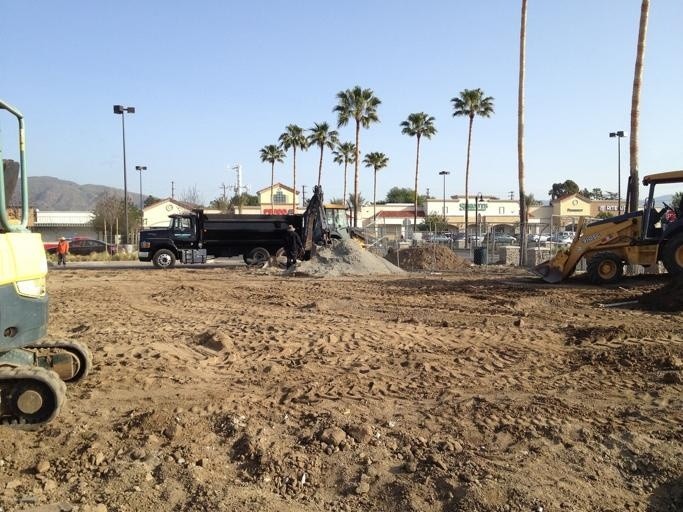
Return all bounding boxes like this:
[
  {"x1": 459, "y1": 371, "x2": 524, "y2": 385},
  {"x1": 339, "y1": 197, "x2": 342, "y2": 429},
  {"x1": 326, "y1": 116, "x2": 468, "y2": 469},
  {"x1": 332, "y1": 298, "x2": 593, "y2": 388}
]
[
  {"x1": 531, "y1": 170, "x2": 683, "y2": 284},
  {"x1": 0, "y1": 98, "x2": 92, "y2": 429}
]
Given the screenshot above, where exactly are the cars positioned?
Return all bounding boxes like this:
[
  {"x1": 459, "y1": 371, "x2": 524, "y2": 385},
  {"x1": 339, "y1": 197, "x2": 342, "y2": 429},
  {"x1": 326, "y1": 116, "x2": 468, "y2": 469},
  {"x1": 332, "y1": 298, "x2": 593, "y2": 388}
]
[
  {"x1": 530, "y1": 235, "x2": 551, "y2": 242},
  {"x1": 429, "y1": 236, "x2": 454, "y2": 243},
  {"x1": 47, "y1": 238, "x2": 116, "y2": 255},
  {"x1": 484, "y1": 232, "x2": 516, "y2": 244}
]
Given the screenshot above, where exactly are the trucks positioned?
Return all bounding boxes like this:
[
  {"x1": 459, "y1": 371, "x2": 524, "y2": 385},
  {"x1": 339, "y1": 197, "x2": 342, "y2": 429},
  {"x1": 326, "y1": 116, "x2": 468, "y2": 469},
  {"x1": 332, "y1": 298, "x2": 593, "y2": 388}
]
[{"x1": 139, "y1": 207, "x2": 303, "y2": 268}]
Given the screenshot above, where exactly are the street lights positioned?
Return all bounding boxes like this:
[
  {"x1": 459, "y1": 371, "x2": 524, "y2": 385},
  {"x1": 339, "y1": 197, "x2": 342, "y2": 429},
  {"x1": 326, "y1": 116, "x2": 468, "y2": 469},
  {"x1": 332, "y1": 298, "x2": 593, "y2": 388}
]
[
  {"x1": 474, "y1": 190, "x2": 483, "y2": 246},
  {"x1": 438, "y1": 170, "x2": 450, "y2": 224},
  {"x1": 113, "y1": 106, "x2": 135, "y2": 246},
  {"x1": 136, "y1": 165, "x2": 147, "y2": 227},
  {"x1": 609, "y1": 131, "x2": 628, "y2": 215}
]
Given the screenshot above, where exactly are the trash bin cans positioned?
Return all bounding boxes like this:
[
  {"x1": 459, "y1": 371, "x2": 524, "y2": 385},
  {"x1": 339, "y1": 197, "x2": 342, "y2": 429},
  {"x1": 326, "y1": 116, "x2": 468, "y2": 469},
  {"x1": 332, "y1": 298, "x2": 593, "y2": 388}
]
[{"x1": 474, "y1": 247, "x2": 487, "y2": 265}]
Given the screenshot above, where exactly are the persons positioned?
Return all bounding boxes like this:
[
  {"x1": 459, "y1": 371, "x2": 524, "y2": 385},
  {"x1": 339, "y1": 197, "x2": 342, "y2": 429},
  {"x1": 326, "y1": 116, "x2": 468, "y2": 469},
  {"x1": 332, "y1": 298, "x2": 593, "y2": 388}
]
[
  {"x1": 282, "y1": 224, "x2": 305, "y2": 271},
  {"x1": 55, "y1": 237, "x2": 69, "y2": 266}
]
